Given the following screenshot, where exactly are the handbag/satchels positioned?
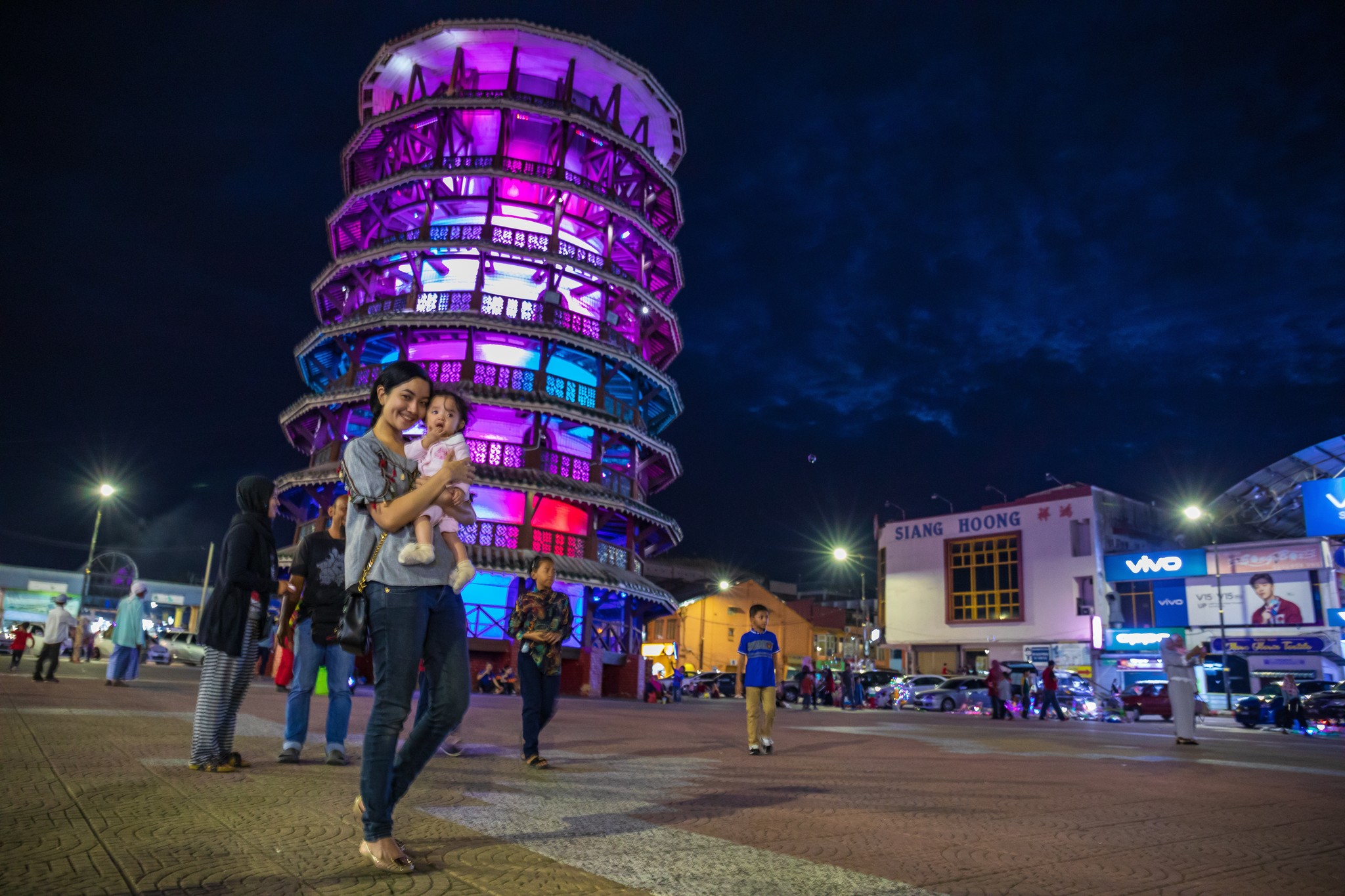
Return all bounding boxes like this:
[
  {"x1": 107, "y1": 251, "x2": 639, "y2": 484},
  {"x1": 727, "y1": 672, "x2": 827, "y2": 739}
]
[{"x1": 337, "y1": 585, "x2": 372, "y2": 659}]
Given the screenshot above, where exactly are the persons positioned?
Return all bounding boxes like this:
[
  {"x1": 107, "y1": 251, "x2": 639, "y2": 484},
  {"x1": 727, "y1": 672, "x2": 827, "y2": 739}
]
[
  {"x1": 334, "y1": 360, "x2": 477, "y2": 874},
  {"x1": 9, "y1": 622, "x2": 35, "y2": 671},
  {"x1": 670, "y1": 661, "x2": 686, "y2": 704},
  {"x1": 398, "y1": 388, "x2": 476, "y2": 595},
  {"x1": 255, "y1": 615, "x2": 294, "y2": 693},
  {"x1": 981, "y1": 659, "x2": 1015, "y2": 721},
  {"x1": 105, "y1": 582, "x2": 146, "y2": 687},
  {"x1": 1039, "y1": 660, "x2": 1070, "y2": 722},
  {"x1": 496, "y1": 665, "x2": 517, "y2": 695},
  {"x1": 1111, "y1": 678, "x2": 1122, "y2": 693},
  {"x1": 840, "y1": 663, "x2": 865, "y2": 710},
  {"x1": 822, "y1": 668, "x2": 835, "y2": 706},
  {"x1": 735, "y1": 604, "x2": 784, "y2": 755},
  {"x1": 1249, "y1": 573, "x2": 1303, "y2": 625},
  {"x1": 33, "y1": 594, "x2": 77, "y2": 683},
  {"x1": 506, "y1": 556, "x2": 574, "y2": 770},
  {"x1": 800, "y1": 655, "x2": 820, "y2": 711},
  {"x1": 188, "y1": 475, "x2": 296, "y2": 773},
  {"x1": 942, "y1": 663, "x2": 978, "y2": 678},
  {"x1": 666, "y1": 678, "x2": 720, "y2": 699},
  {"x1": 276, "y1": 495, "x2": 355, "y2": 765},
  {"x1": 1282, "y1": 674, "x2": 1309, "y2": 730},
  {"x1": 1021, "y1": 668, "x2": 1032, "y2": 719},
  {"x1": 877, "y1": 692, "x2": 895, "y2": 709},
  {"x1": 1159, "y1": 633, "x2": 1205, "y2": 745},
  {"x1": 70, "y1": 617, "x2": 93, "y2": 664},
  {"x1": 477, "y1": 661, "x2": 503, "y2": 695}
]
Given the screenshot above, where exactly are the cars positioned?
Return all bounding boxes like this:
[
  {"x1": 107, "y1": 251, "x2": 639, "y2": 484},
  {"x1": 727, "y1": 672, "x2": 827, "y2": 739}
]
[
  {"x1": 0, "y1": 620, "x2": 170, "y2": 665},
  {"x1": 656, "y1": 659, "x2": 1120, "y2": 711},
  {"x1": 1231, "y1": 680, "x2": 1345, "y2": 729},
  {"x1": 1101, "y1": 680, "x2": 1206, "y2": 723},
  {"x1": 153, "y1": 629, "x2": 206, "y2": 666}
]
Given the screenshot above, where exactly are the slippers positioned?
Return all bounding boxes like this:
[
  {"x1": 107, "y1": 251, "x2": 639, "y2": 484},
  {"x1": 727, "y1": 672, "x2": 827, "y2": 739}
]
[{"x1": 526, "y1": 755, "x2": 549, "y2": 770}]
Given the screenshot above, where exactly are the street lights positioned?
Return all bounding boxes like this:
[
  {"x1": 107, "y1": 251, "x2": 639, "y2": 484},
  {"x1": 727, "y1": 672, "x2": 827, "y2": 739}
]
[
  {"x1": 1181, "y1": 504, "x2": 1232, "y2": 711},
  {"x1": 698, "y1": 579, "x2": 730, "y2": 671},
  {"x1": 86, "y1": 484, "x2": 114, "y2": 569},
  {"x1": 832, "y1": 545, "x2": 868, "y2": 666}
]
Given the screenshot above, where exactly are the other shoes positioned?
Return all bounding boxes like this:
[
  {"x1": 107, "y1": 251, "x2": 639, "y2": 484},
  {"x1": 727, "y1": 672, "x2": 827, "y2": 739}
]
[
  {"x1": 325, "y1": 749, "x2": 348, "y2": 765},
  {"x1": 189, "y1": 759, "x2": 234, "y2": 772},
  {"x1": 32, "y1": 673, "x2": 43, "y2": 683},
  {"x1": 440, "y1": 741, "x2": 462, "y2": 757},
  {"x1": 1176, "y1": 737, "x2": 1199, "y2": 745},
  {"x1": 226, "y1": 751, "x2": 251, "y2": 766},
  {"x1": 44, "y1": 675, "x2": 59, "y2": 684},
  {"x1": 275, "y1": 684, "x2": 290, "y2": 694},
  {"x1": 277, "y1": 748, "x2": 299, "y2": 763},
  {"x1": 113, "y1": 679, "x2": 130, "y2": 688},
  {"x1": 105, "y1": 680, "x2": 112, "y2": 686}
]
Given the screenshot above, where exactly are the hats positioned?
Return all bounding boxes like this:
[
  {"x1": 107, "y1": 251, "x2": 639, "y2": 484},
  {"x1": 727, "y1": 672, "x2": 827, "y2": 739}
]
[
  {"x1": 130, "y1": 580, "x2": 147, "y2": 595},
  {"x1": 54, "y1": 594, "x2": 67, "y2": 603}
]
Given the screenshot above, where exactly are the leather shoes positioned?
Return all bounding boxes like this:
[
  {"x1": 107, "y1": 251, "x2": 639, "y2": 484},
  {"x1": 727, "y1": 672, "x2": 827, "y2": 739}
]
[
  {"x1": 352, "y1": 794, "x2": 408, "y2": 851},
  {"x1": 358, "y1": 838, "x2": 414, "y2": 873}
]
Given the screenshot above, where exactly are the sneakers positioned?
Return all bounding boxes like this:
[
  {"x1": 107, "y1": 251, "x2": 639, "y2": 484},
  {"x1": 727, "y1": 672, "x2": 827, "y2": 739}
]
[
  {"x1": 760, "y1": 736, "x2": 774, "y2": 754},
  {"x1": 748, "y1": 744, "x2": 760, "y2": 755}
]
